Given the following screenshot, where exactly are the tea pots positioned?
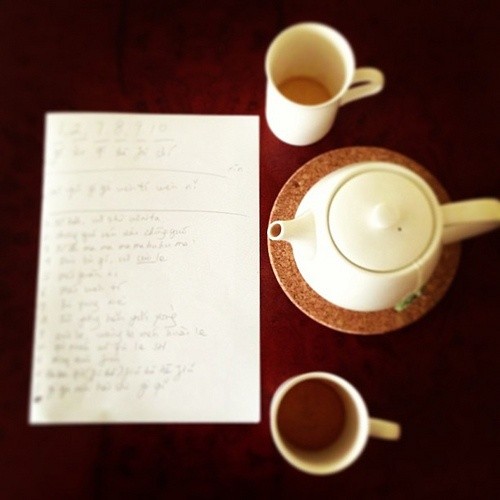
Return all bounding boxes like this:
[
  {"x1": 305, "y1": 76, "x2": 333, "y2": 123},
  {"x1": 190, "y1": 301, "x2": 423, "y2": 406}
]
[{"x1": 265, "y1": 148, "x2": 500, "y2": 336}]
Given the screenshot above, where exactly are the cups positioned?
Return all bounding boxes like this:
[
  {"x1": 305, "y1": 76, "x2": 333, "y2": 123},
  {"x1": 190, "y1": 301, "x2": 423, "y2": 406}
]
[
  {"x1": 270, "y1": 371, "x2": 402, "y2": 476},
  {"x1": 265, "y1": 22, "x2": 386, "y2": 146}
]
